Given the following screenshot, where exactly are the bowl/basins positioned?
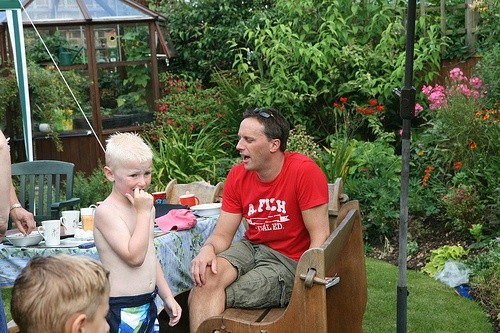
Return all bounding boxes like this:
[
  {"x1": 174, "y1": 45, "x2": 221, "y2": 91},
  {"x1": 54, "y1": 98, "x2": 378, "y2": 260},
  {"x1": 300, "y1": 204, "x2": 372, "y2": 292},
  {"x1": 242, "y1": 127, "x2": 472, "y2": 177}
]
[
  {"x1": 189, "y1": 203, "x2": 222, "y2": 217},
  {"x1": 5, "y1": 233, "x2": 44, "y2": 246}
]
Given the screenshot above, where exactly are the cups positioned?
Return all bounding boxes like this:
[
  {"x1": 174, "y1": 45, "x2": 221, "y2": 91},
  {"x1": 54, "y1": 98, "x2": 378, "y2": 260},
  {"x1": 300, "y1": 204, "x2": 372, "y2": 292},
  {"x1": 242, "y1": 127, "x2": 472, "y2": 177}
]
[
  {"x1": 38, "y1": 201, "x2": 104, "y2": 246},
  {"x1": 152, "y1": 192, "x2": 166, "y2": 204},
  {"x1": 179, "y1": 194, "x2": 200, "y2": 207}
]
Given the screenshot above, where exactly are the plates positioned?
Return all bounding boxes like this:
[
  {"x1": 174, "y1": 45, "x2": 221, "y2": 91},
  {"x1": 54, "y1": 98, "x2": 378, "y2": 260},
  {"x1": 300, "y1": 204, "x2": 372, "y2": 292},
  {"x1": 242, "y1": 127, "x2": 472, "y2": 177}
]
[{"x1": 60, "y1": 226, "x2": 80, "y2": 238}]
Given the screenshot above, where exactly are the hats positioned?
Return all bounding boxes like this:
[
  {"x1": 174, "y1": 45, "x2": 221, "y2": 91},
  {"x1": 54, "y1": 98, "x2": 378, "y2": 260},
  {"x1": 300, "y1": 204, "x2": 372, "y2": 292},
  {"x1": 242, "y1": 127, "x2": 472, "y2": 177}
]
[{"x1": 155, "y1": 209, "x2": 197, "y2": 232}]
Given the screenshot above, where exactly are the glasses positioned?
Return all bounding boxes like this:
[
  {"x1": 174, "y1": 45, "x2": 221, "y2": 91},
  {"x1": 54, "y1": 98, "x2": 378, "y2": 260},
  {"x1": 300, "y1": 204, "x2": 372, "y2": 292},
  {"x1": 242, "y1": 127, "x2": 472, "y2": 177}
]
[{"x1": 254, "y1": 107, "x2": 284, "y2": 132}]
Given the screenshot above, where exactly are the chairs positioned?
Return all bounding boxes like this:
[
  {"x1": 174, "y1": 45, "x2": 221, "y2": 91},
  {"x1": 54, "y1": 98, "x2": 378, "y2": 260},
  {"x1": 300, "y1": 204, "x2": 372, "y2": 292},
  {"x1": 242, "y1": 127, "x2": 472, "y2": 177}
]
[
  {"x1": 328, "y1": 177, "x2": 349, "y2": 212},
  {"x1": 165, "y1": 179, "x2": 223, "y2": 207},
  {"x1": 7, "y1": 160, "x2": 80, "y2": 230}
]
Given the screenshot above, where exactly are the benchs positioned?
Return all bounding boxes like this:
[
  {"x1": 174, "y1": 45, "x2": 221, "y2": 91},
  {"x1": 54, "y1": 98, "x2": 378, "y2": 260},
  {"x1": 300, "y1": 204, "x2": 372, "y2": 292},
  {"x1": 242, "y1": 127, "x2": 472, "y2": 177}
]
[{"x1": 194, "y1": 200, "x2": 368, "y2": 333}]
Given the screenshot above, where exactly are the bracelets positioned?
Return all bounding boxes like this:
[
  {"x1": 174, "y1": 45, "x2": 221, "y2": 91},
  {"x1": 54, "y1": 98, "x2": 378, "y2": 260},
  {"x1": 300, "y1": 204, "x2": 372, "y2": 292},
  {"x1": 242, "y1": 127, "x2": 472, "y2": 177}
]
[{"x1": 10, "y1": 203, "x2": 22, "y2": 210}]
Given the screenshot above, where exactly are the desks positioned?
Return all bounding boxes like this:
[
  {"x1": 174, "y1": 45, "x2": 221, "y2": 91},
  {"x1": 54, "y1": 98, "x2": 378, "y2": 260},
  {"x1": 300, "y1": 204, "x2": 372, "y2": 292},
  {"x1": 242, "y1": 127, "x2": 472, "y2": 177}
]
[{"x1": 0, "y1": 212, "x2": 248, "y2": 318}]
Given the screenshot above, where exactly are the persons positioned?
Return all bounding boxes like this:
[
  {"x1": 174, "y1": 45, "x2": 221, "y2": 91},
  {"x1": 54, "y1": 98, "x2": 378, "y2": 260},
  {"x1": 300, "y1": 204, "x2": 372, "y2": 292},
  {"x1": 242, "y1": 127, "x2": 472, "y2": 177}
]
[
  {"x1": 0, "y1": 129, "x2": 37, "y2": 333},
  {"x1": 186, "y1": 106, "x2": 332, "y2": 333},
  {"x1": 10, "y1": 251, "x2": 111, "y2": 333},
  {"x1": 93, "y1": 132, "x2": 183, "y2": 333}
]
